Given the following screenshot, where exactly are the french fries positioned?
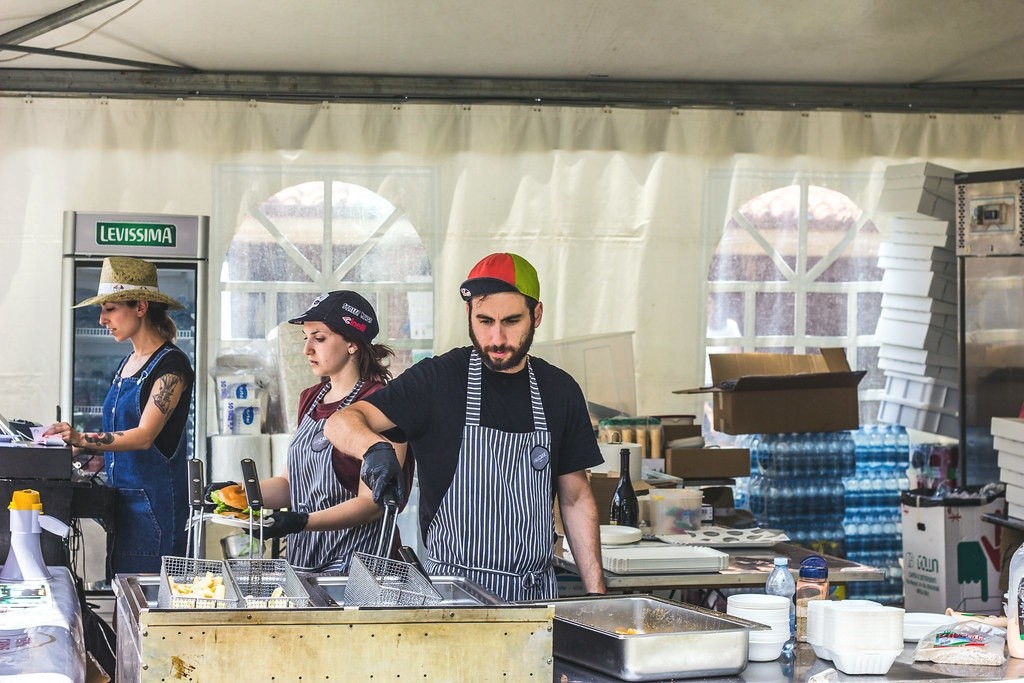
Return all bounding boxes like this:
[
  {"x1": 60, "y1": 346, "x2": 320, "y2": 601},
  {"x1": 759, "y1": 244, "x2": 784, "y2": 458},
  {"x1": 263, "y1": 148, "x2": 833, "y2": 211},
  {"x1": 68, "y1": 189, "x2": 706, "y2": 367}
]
[
  {"x1": 169, "y1": 569, "x2": 227, "y2": 601},
  {"x1": 246, "y1": 587, "x2": 283, "y2": 598}
]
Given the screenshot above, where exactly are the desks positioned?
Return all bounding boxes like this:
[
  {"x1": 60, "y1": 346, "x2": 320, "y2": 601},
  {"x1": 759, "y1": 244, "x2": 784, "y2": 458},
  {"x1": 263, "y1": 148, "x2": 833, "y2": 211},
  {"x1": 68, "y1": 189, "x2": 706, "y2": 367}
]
[{"x1": 552, "y1": 535, "x2": 886, "y2": 612}]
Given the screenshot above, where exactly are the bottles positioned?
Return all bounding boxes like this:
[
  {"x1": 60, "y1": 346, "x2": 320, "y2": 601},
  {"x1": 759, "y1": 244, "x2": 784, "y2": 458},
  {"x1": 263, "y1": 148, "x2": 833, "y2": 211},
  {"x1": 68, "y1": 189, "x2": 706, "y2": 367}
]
[
  {"x1": 610, "y1": 449, "x2": 638, "y2": 529},
  {"x1": 730, "y1": 425, "x2": 909, "y2": 606},
  {"x1": 796, "y1": 557, "x2": 829, "y2": 642},
  {"x1": 598, "y1": 417, "x2": 662, "y2": 461},
  {"x1": 766, "y1": 558, "x2": 799, "y2": 653},
  {"x1": 1006, "y1": 543, "x2": 1024, "y2": 658}
]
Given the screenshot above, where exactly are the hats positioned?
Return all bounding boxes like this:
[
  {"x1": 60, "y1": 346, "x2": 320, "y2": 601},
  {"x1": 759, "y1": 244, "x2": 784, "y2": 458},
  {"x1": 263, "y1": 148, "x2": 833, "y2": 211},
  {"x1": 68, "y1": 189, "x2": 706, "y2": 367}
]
[
  {"x1": 459, "y1": 253, "x2": 541, "y2": 303},
  {"x1": 288, "y1": 289, "x2": 380, "y2": 344},
  {"x1": 70, "y1": 258, "x2": 187, "y2": 311}
]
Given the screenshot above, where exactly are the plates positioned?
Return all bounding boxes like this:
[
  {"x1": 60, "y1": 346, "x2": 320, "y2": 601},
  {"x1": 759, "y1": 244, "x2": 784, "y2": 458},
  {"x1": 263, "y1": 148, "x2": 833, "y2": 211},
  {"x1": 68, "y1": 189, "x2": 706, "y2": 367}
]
[
  {"x1": 902, "y1": 612, "x2": 952, "y2": 644},
  {"x1": 599, "y1": 524, "x2": 642, "y2": 545},
  {"x1": 210, "y1": 517, "x2": 261, "y2": 530}
]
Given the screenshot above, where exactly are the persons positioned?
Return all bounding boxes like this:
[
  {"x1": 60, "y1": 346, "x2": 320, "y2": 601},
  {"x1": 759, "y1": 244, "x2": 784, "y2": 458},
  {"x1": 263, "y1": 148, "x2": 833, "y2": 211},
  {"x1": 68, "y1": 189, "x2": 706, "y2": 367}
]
[
  {"x1": 204, "y1": 292, "x2": 415, "y2": 580},
  {"x1": 41, "y1": 258, "x2": 195, "y2": 579},
  {"x1": 324, "y1": 253, "x2": 606, "y2": 603}
]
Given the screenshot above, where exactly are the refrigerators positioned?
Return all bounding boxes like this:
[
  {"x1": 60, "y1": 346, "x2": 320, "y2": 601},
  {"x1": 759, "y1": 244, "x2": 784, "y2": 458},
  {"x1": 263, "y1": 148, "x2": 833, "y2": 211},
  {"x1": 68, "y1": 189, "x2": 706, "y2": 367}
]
[{"x1": 57, "y1": 210, "x2": 210, "y2": 623}]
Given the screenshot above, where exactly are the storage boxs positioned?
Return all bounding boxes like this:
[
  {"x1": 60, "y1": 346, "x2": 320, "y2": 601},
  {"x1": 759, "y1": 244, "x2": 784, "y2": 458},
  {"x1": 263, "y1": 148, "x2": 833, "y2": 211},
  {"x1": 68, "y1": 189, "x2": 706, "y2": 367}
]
[
  {"x1": 807, "y1": 162, "x2": 1024, "y2": 675},
  {"x1": 553, "y1": 470, "x2": 622, "y2": 534},
  {"x1": 669, "y1": 347, "x2": 867, "y2": 436},
  {"x1": 665, "y1": 447, "x2": 752, "y2": 479}
]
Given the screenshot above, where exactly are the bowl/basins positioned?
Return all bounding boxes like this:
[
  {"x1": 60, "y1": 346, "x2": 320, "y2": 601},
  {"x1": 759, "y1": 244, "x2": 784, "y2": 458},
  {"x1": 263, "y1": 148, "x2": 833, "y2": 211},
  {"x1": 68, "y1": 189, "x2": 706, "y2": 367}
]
[
  {"x1": 667, "y1": 435, "x2": 704, "y2": 447},
  {"x1": 651, "y1": 415, "x2": 695, "y2": 425},
  {"x1": 726, "y1": 594, "x2": 791, "y2": 661},
  {"x1": 806, "y1": 599, "x2": 907, "y2": 674}
]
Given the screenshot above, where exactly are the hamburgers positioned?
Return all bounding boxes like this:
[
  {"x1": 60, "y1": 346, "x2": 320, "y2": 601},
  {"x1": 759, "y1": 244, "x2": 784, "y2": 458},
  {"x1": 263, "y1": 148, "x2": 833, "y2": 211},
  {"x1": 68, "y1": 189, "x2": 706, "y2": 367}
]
[{"x1": 211, "y1": 485, "x2": 260, "y2": 520}]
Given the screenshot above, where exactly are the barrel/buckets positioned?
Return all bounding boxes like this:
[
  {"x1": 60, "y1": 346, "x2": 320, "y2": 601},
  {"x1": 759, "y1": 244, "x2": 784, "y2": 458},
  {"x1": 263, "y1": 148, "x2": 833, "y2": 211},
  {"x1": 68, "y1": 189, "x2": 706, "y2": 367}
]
[{"x1": 650, "y1": 489, "x2": 705, "y2": 534}]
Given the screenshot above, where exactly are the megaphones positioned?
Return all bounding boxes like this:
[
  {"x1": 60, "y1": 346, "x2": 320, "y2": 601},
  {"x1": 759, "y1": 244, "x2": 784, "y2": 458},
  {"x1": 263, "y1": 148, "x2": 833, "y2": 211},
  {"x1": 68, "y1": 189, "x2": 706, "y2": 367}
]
[{"x1": 0, "y1": 489, "x2": 70, "y2": 581}]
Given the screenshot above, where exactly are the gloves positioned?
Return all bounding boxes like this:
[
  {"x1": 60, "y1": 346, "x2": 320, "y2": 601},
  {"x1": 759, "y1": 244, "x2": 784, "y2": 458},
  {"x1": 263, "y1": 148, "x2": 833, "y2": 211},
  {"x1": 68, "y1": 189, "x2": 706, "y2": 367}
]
[
  {"x1": 362, "y1": 441, "x2": 405, "y2": 512},
  {"x1": 243, "y1": 510, "x2": 309, "y2": 542},
  {"x1": 205, "y1": 480, "x2": 238, "y2": 503}
]
